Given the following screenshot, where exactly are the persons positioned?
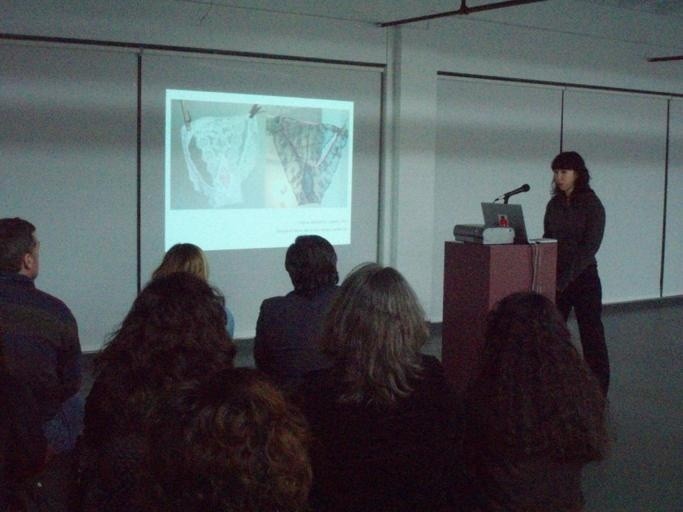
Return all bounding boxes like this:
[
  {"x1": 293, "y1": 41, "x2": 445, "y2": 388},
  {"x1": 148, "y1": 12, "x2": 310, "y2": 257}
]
[
  {"x1": 71, "y1": 271, "x2": 235, "y2": 511},
  {"x1": 101, "y1": 368, "x2": 318, "y2": 511},
  {"x1": 542, "y1": 151, "x2": 609, "y2": 396},
  {"x1": 151, "y1": 243, "x2": 233, "y2": 343},
  {"x1": 252, "y1": 236, "x2": 340, "y2": 381},
  {"x1": 273, "y1": 263, "x2": 463, "y2": 511},
  {"x1": 462, "y1": 292, "x2": 611, "y2": 512},
  {"x1": 1, "y1": 217, "x2": 82, "y2": 511}
]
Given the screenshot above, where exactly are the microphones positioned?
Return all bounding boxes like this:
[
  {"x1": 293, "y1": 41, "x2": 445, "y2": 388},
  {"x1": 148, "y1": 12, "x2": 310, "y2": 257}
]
[{"x1": 495, "y1": 184, "x2": 530, "y2": 201}]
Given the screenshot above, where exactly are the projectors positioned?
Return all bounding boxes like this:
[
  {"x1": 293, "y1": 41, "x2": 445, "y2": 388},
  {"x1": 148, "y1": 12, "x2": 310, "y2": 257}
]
[{"x1": 454, "y1": 225, "x2": 516, "y2": 244}]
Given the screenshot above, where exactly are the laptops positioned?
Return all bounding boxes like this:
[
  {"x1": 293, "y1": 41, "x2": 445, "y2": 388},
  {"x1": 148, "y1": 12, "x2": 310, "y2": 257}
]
[{"x1": 480, "y1": 202, "x2": 531, "y2": 245}]
